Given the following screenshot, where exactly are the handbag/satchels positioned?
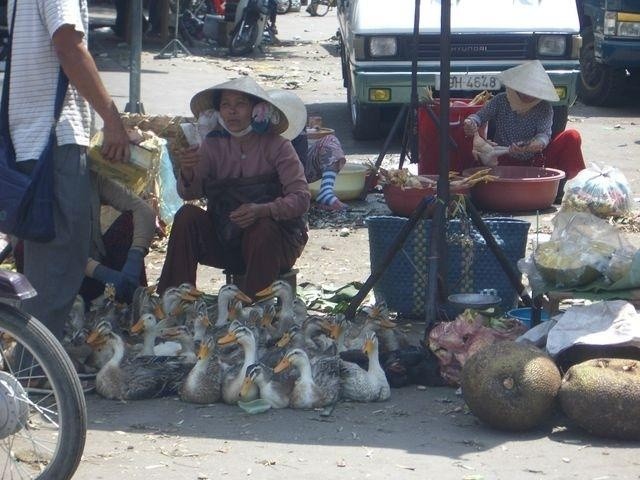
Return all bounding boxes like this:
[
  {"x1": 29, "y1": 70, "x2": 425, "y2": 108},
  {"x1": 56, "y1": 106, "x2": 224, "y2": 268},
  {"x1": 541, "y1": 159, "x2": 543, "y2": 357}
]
[{"x1": 0, "y1": 125, "x2": 57, "y2": 243}]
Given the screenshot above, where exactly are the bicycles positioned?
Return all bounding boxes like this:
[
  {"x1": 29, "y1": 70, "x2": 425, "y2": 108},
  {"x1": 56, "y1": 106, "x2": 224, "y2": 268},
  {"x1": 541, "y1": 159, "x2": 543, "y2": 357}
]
[{"x1": 313, "y1": 0, "x2": 332, "y2": 18}]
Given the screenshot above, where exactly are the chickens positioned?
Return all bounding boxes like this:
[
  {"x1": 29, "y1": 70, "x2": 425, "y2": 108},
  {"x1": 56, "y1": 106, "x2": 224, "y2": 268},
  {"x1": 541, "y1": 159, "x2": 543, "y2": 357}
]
[
  {"x1": 421, "y1": 84, "x2": 493, "y2": 107},
  {"x1": 362, "y1": 157, "x2": 500, "y2": 193},
  {"x1": 464, "y1": 118, "x2": 545, "y2": 168}
]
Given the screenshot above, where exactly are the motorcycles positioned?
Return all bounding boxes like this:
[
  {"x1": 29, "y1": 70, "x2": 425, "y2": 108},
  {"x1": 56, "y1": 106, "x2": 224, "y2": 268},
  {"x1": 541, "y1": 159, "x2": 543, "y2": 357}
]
[
  {"x1": 276, "y1": 0, "x2": 292, "y2": 16},
  {"x1": 231, "y1": 0, "x2": 266, "y2": 55},
  {"x1": 0, "y1": 240, "x2": 88, "y2": 480}
]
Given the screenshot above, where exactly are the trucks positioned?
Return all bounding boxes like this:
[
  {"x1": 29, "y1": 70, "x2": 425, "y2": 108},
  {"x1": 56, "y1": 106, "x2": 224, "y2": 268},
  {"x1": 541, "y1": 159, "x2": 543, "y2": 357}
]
[{"x1": 576, "y1": 0, "x2": 639, "y2": 106}]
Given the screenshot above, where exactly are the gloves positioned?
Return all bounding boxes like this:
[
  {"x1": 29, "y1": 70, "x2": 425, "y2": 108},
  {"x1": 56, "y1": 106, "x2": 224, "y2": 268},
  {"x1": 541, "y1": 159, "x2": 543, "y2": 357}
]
[
  {"x1": 93, "y1": 264, "x2": 139, "y2": 298},
  {"x1": 121, "y1": 250, "x2": 144, "y2": 280}
]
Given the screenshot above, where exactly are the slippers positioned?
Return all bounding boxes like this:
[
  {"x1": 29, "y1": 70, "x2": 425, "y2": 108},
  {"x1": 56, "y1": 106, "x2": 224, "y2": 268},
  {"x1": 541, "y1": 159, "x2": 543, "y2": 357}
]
[{"x1": 313, "y1": 198, "x2": 343, "y2": 212}]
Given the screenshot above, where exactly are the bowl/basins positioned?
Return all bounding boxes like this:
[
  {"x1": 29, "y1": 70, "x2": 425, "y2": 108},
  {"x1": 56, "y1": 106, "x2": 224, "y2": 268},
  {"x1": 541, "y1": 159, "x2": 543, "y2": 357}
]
[
  {"x1": 532, "y1": 239, "x2": 617, "y2": 288},
  {"x1": 382, "y1": 174, "x2": 465, "y2": 220},
  {"x1": 508, "y1": 305, "x2": 562, "y2": 328},
  {"x1": 463, "y1": 165, "x2": 565, "y2": 212},
  {"x1": 308, "y1": 164, "x2": 368, "y2": 201}
]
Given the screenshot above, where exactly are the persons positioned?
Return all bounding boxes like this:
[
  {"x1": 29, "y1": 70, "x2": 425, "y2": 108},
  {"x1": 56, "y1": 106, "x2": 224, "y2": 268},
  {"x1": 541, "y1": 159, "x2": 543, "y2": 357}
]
[
  {"x1": 3, "y1": 0, "x2": 134, "y2": 397},
  {"x1": 12, "y1": 168, "x2": 155, "y2": 306},
  {"x1": 263, "y1": 88, "x2": 349, "y2": 212},
  {"x1": 155, "y1": 75, "x2": 312, "y2": 307},
  {"x1": 463, "y1": 59, "x2": 586, "y2": 180}
]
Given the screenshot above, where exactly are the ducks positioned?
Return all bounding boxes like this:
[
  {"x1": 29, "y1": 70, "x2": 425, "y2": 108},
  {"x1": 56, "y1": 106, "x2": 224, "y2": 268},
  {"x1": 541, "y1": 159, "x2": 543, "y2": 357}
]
[{"x1": 61, "y1": 278, "x2": 443, "y2": 409}]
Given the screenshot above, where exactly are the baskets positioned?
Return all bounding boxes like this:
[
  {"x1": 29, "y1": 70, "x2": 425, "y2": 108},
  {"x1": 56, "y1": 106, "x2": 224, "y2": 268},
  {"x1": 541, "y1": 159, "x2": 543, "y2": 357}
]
[{"x1": 364, "y1": 215, "x2": 531, "y2": 321}]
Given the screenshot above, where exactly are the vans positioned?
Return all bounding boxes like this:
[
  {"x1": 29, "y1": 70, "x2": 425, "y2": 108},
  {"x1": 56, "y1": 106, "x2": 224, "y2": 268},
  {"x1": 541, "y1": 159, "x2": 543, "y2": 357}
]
[{"x1": 336, "y1": 0, "x2": 582, "y2": 140}]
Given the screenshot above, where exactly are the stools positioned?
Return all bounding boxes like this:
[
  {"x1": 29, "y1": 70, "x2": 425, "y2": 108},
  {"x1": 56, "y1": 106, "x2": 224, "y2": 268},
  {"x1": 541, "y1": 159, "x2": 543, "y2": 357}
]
[{"x1": 223, "y1": 270, "x2": 300, "y2": 306}]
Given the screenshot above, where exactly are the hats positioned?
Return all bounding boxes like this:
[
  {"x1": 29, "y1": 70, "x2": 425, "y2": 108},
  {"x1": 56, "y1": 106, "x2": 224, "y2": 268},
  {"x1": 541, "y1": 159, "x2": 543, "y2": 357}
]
[
  {"x1": 265, "y1": 90, "x2": 308, "y2": 141},
  {"x1": 190, "y1": 77, "x2": 289, "y2": 134},
  {"x1": 495, "y1": 60, "x2": 560, "y2": 102}
]
